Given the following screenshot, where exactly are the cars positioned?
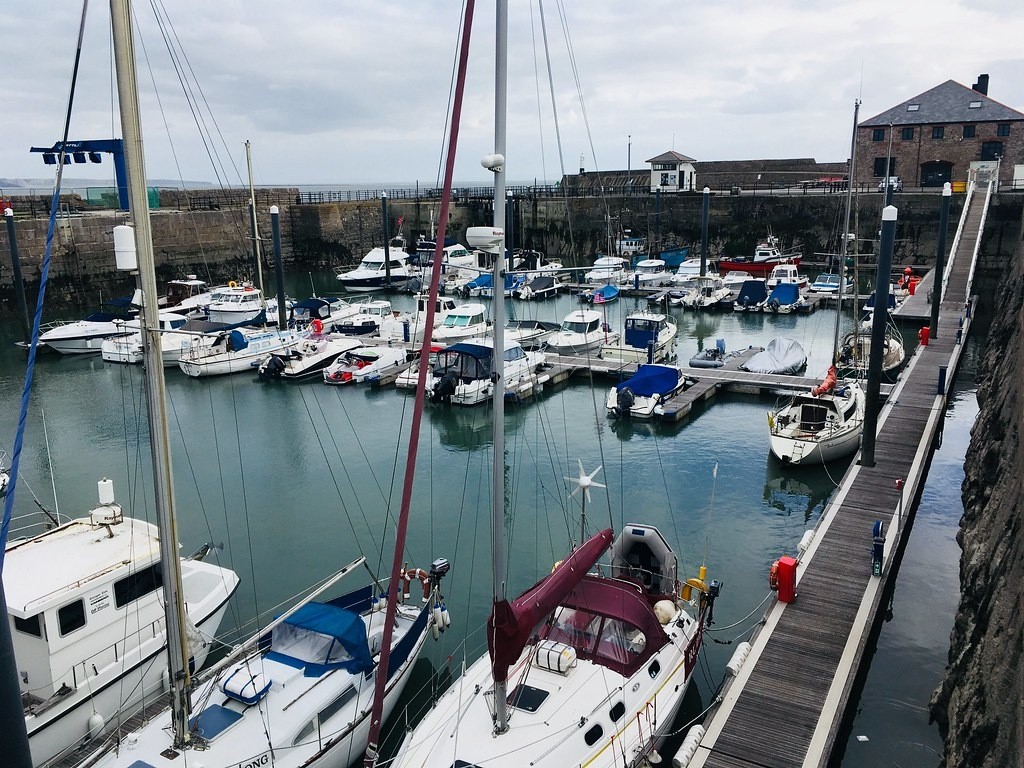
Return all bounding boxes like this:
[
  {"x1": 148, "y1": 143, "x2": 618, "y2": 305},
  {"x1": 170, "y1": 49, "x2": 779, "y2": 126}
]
[{"x1": 878, "y1": 177, "x2": 903, "y2": 193}]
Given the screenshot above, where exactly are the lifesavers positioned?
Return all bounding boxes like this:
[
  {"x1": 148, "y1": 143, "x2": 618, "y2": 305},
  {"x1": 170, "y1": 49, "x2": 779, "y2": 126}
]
[
  {"x1": 403, "y1": 567, "x2": 432, "y2": 603},
  {"x1": 769, "y1": 560, "x2": 780, "y2": 592},
  {"x1": 245, "y1": 287, "x2": 253, "y2": 291},
  {"x1": 228, "y1": 280, "x2": 237, "y2": 287},
  {"x1": 681, "y1": 577, "x2": 709, "y2": 608},
  {"x1": 766, "y1": 410, "x2": 775, "y2": 429}
]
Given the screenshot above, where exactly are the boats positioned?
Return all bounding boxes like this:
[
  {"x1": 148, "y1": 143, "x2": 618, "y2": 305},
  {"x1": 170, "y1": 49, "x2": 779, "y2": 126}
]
[
  {"x1": 15, "y1": 204, "x2": 853, "y2": 419},
  {"x1": 69, "y1": 1, "x2": 451, "y2": 768},
  {"x1": 0, "y1": 408, "x2": 241, "y2": 768}
]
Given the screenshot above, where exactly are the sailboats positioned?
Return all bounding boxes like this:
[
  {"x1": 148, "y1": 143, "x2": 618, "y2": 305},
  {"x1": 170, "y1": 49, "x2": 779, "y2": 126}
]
[
  {"x1": 177, "y1": 140, "x2": 312, "y2": 377},
  {"x1": 364, "y1": 0, "x2": 724, "y2": 768},
  {"x1": 766, "y1": 99, "x2": 866, "y2": 466},
  {"x1": 834, "y1": 158, "x2": 905, "y2": 380}
]
